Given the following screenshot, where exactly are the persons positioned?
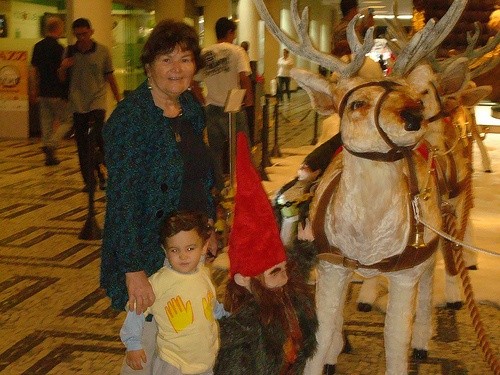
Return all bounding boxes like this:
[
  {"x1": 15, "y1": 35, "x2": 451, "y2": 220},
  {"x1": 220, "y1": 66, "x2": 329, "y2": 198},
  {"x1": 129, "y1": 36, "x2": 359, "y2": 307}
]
[
  {"x1": 247, "y1": 61, "x2": 265, "y2": 148},
  {"x1": 277, "y1": 48, "x2": 294, "y2": 102},
  {"x1": 100, "y1": 18, "x2": 225, "y2": 374},
  {"x1": 57, "y1": 17, "x2": 122, "y2": 191},
  {"x1": 31, "y1": 17, "x2": 74, "y2": 166},
  {"x1": 119, "y1": 209, "x2": 232, "y2": 374},
  {"x1": 333, "y1": 0, "x2": 375, "y2": 56},
  {"x1": 190, "y1": 17, "x2": 254, "y2": 197},
  {"x1": 379, "y1": 55, "x2": 387, "y2": 71}
]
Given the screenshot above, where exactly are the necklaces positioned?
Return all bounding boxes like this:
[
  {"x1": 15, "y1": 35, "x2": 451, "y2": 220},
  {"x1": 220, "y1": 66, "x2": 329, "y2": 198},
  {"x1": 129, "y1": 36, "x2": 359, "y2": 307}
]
[{"x1": 162, "y1": 97, "x2": 183, "y2": 142}]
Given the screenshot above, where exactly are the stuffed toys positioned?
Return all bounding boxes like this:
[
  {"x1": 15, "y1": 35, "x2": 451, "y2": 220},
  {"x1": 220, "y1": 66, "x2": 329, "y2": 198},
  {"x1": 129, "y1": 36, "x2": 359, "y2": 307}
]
[{"x1": 210, "y1": 131, "x2": 319, "y2": 375}]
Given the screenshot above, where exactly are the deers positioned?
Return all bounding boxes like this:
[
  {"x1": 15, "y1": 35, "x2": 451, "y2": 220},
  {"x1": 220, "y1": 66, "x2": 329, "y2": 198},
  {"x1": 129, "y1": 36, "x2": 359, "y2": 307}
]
[{"x1": 252, "y1": 0, "x2": 499, "y2": 375}]
